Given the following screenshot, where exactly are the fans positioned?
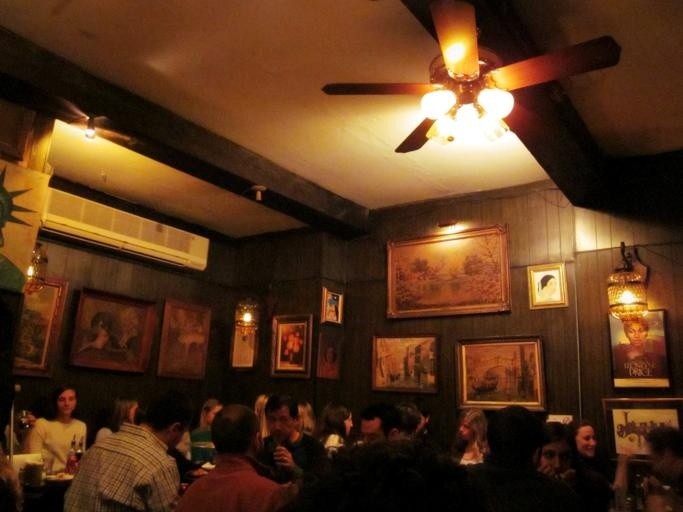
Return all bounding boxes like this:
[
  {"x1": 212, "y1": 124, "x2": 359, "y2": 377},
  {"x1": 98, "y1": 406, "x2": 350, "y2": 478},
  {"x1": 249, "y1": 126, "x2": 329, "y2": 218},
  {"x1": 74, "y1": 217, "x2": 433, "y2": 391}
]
[{"x1": 321, "y1": 0, "x2": 621, "y2": 153}]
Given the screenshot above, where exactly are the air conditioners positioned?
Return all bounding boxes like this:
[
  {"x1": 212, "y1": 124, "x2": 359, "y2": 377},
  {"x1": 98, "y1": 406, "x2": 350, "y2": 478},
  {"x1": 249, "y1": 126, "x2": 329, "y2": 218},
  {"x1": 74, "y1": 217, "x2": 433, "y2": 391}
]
[{"x1": 41, "y1": 185, "x2": 210, "y2": 272}]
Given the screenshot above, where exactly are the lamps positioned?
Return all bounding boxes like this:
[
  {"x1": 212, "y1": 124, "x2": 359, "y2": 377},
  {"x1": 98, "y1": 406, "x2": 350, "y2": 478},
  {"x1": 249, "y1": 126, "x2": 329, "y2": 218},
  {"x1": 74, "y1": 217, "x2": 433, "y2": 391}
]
[
  {"x1": 84, "y1": 115, "x2": 96, "y2": 140},
  {"x1": 421, "y1": 82, "x2": 514, "y2": 144},
  {"x1": 607, "y1": 243, "x2": 648, "y2": 322},
  {"x1": 26, "y1": 242, "x2": 48, "y2": 295}
]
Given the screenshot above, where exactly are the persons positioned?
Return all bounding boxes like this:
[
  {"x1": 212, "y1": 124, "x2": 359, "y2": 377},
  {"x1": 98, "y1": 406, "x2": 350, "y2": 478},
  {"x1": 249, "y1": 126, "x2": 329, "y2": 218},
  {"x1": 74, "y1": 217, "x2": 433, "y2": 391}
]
[
  {"x1": 538, "y1": 273, "x2": 559, "y2": 301},
  {"x1": 0, "y1": 379, "x2": 682, "y2": 512},
  {"x1": 612, "y1": 314, "x2": 669, "y2": 378},
  {"x1": 280, "y1": 329, "x2": 305, "y2": 365},
  {"x1": 316, "y1": 341, "x2": 342, "y2": 379}
]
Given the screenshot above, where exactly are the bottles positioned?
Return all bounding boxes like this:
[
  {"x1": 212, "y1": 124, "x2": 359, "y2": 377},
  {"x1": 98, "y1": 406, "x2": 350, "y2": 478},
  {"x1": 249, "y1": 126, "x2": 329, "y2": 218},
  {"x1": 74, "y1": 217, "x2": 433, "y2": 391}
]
[{"x1": 68, "y1": 434, "x2": 84, "y2": 473}]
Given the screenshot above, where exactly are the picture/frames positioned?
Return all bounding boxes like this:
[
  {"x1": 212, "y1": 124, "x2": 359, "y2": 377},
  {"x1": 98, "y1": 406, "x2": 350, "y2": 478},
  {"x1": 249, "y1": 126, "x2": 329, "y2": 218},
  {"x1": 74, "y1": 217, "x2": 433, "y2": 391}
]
[
  {"x1": 371, "y1": 224, "x2": 569, "y2": 410},
  {"x1": 607, "y1": 309, "x2": 671, "y2": 393},
  {"x1": 601, "y1": 398, "x2": 683, "y2": 464}
]
